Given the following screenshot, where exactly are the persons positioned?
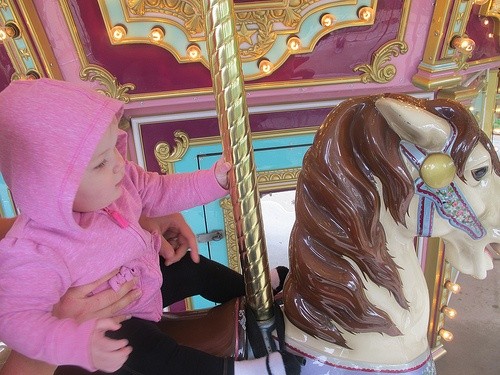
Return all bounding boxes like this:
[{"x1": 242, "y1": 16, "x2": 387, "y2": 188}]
[
  {"x1": 0, "y1": 78, "x2": 290, "y2": 375},
  {"x1": 2, "y1": 210, "x2": 199, "y2": 375}
]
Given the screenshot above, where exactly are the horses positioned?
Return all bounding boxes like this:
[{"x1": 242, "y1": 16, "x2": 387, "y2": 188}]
[{"x1": 1, "y1": 92, "x2": 497, "y2": 375}]
